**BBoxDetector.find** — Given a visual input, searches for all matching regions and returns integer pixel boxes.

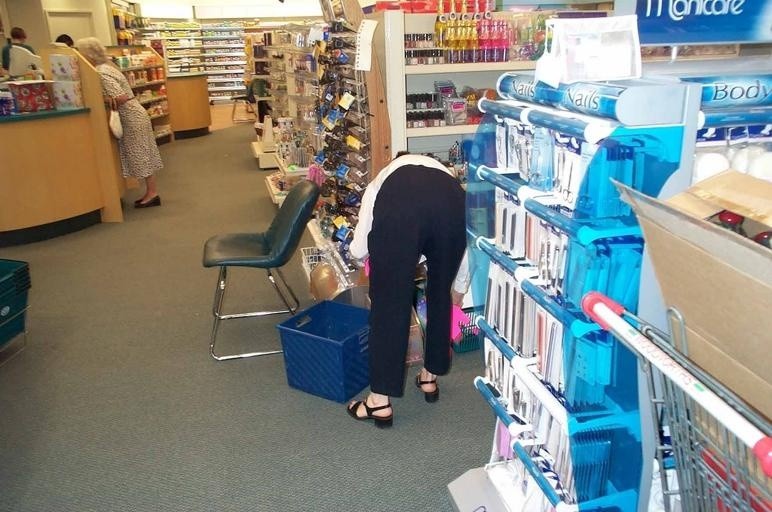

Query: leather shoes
[134,194,160,209]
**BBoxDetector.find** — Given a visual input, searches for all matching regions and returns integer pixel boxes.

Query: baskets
[0,258,32,346]
[300,246,323,282]
[451,305,484,354]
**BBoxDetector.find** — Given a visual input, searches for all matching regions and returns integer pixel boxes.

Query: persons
[1,27,38,70]
[56,34,74,48]
[342,148,468,430]
[74,37,165,208]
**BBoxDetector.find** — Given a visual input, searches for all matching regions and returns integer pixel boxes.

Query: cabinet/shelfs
[110,1,245,148]
[252,0,391,274]
[446,71,703,512]
[365,11,636,193]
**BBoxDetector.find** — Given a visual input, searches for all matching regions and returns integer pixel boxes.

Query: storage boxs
[609,166,772,492]
[2,80,58,113]
[364,293,424,368]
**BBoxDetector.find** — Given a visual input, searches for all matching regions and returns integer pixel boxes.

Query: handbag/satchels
[108,110,123,139]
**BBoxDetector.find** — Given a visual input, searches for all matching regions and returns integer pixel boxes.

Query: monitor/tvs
[9,45,41,76]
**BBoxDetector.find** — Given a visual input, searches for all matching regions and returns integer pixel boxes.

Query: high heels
[415,372,440,403]
[347,398,394,430]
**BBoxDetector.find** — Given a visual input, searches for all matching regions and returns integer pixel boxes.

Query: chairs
[201,179,321,362]
[230,76,257,121]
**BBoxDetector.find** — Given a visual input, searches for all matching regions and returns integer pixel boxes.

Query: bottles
[437,20,510,63]
